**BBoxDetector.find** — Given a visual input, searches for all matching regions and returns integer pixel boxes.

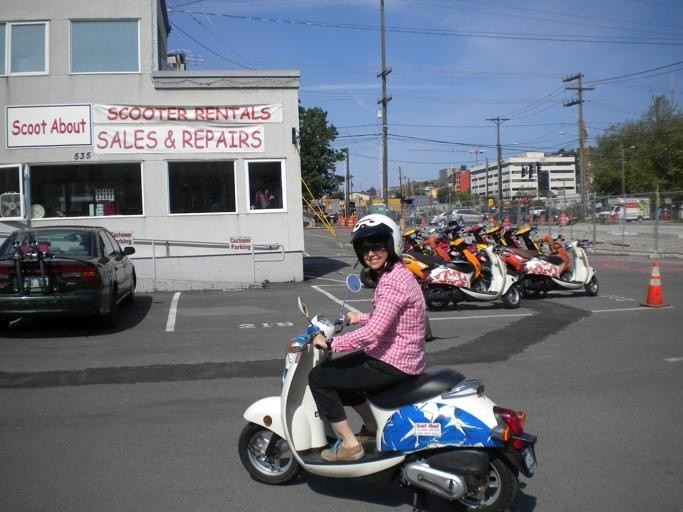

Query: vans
[595,205,642,222]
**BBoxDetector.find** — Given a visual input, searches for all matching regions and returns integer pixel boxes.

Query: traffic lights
[537,163,541,173]
[529,165,533,179]
[521,167,526,177]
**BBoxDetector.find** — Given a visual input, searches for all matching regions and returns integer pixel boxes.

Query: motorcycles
[237,275,538,512]
[359,214,599,309]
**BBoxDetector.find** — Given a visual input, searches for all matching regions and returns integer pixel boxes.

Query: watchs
[324,336,333,350]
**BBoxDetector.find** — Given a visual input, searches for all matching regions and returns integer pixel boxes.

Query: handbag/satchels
[426,316,432,341]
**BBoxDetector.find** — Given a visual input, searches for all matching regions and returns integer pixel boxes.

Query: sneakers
[321,424,376,462]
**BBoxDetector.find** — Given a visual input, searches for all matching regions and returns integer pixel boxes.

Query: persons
[304,211,431,463]
[263,188,275,209]
[250,178,270,210]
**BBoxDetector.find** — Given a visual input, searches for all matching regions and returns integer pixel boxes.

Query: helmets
[351,213,402,268]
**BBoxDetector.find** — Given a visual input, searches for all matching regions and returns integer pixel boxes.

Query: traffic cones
[639,260,670,308]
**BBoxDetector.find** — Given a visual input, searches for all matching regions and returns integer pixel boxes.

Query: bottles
[88,199,118,216]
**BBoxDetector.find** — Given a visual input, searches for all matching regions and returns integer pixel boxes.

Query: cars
[430,212,449,228]
[449,209,487,224]
[529,206,545,216]
[0,225,136,329]
